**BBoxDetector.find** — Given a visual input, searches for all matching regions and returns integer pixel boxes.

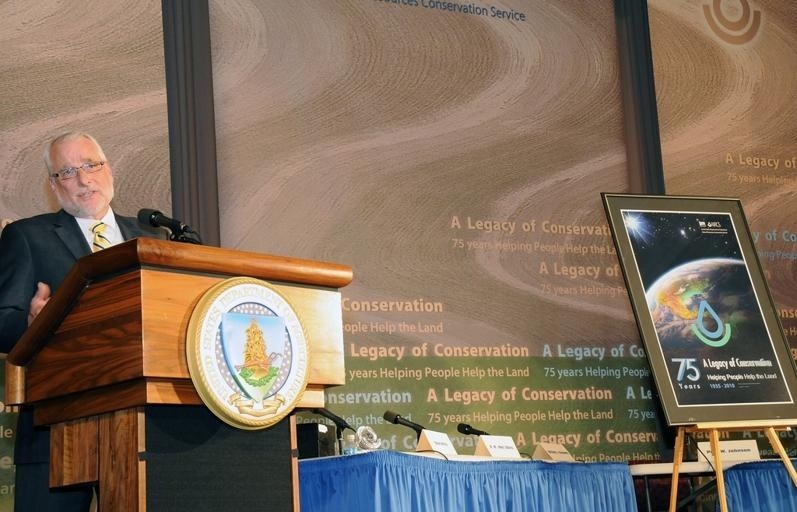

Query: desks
[629,458,796,511]
[298,451,636,512]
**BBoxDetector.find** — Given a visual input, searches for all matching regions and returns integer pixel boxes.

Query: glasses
[51,162,106,180]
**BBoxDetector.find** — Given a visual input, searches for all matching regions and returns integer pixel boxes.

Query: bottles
[343,434,358,456]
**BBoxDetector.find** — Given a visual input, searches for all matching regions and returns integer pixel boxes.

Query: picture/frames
[602,192,795,425]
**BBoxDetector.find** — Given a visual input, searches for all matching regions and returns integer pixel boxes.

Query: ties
[89,220,112,253]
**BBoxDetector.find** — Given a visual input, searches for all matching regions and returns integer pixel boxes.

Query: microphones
[383,411,426,433]
[457,423,491,436]
[309,408,351,429]
[137,209,193,233]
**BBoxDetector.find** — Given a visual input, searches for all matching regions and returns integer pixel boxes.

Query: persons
[0,132,169,511]
